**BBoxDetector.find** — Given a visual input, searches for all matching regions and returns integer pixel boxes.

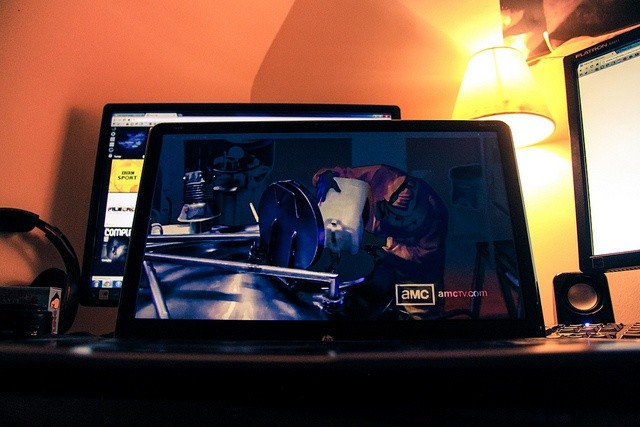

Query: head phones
[1,207,81,335]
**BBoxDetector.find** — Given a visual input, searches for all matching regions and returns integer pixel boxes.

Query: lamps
[451,44,558,146]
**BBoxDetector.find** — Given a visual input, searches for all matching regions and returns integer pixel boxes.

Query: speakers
[553,273,614,325]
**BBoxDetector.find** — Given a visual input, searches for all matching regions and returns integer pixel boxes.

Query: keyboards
[546,322,640,341]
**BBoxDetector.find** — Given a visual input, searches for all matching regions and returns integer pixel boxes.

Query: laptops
[94,120,545,350]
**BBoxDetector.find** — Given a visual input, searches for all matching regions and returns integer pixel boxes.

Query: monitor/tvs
[81,102,400,307]
[563,24,639,273]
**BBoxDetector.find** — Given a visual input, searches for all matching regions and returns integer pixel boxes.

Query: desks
[1,325,603,426]
